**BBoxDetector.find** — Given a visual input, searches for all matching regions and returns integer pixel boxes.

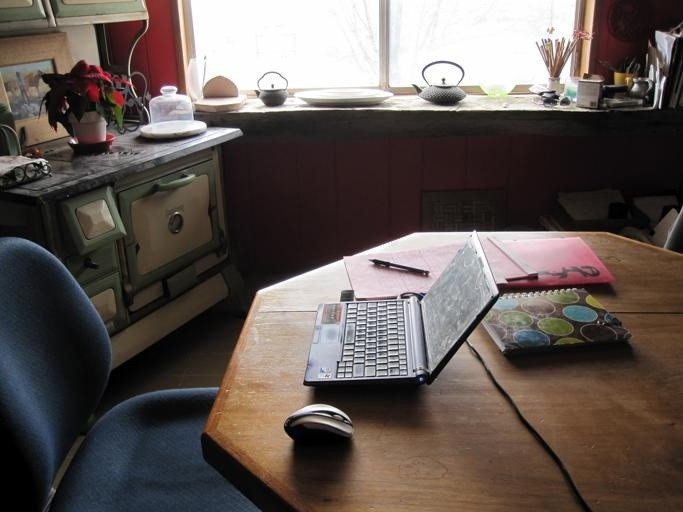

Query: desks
[201,230,682,511]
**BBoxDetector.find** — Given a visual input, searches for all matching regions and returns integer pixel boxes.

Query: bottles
[0,103,19,157]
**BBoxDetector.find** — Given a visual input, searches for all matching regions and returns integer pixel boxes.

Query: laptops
[303,230,500,386]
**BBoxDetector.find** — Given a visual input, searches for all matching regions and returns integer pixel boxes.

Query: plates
[292,87,395,107]
[137,120,207,140]
[66,134,116,153]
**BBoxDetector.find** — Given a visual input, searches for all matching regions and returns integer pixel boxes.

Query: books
[646,29,683,110]
[481,287,632,356]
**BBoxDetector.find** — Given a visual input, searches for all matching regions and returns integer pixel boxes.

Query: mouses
[283,403,354,441]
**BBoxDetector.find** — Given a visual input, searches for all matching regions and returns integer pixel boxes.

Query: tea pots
[253,71,288,108]
[412,60,467,105]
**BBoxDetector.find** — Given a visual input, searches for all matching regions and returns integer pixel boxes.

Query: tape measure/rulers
[487,235,539,276]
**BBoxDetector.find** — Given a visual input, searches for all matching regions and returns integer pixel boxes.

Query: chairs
[0,238,263,510]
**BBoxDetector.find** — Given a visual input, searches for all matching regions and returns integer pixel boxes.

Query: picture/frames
[0,32,82,148]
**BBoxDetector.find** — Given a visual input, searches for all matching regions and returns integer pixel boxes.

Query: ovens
[113,146,248,351]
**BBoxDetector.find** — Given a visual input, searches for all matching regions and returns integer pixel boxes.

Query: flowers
[39,59,133,133]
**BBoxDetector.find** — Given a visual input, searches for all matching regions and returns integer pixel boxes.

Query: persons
[15,71,30,103]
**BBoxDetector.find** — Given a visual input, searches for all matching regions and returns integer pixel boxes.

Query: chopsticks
[535,36,580,77]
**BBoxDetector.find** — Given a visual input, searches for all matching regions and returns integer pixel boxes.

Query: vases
[67,109,107,144]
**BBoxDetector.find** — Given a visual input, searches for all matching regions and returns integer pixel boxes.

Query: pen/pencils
[598,55,641,74]
[536,37,580,77]
[368,259,430,276]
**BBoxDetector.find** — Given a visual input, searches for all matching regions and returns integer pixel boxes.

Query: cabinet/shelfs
[0,0,152,32]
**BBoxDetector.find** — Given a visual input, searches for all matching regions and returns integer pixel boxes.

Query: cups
[614,71,639,91]
[630,76,654,98]
[547,76,559,90]
